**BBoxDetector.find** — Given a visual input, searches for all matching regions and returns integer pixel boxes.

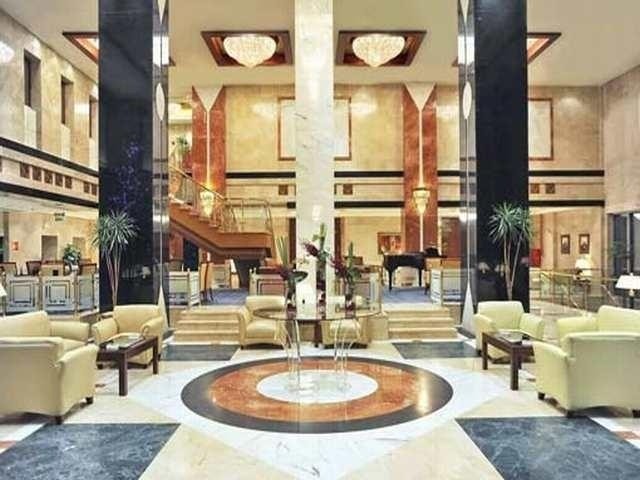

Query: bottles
[100,343,128,351]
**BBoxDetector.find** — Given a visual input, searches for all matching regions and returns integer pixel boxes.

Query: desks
[253,303,382,396]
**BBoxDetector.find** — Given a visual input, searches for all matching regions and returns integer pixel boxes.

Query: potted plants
[335,223,362,312]
[272,232,308,311]
[299,223,334,299]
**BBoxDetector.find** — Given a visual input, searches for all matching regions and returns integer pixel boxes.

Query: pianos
[380,247,446,292]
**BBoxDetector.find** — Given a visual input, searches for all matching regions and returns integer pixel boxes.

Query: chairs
[473,300,545,363]
[237,295,288,350]
[198,262,208,301]
[529,333,640,419]
[319,295,371,349]
[0,310,98,426]
[1,258,97,284]
[90,304,165,369]
[556,304,640,347]
[423,257,462,295]
[205,262,215,302]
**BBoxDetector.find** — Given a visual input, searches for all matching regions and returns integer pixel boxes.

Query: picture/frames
[578,233,589,253]
[377,232,402,254]
[561,235,570,254]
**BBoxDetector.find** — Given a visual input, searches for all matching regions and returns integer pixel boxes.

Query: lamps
[351,33,405,69]
[411,186,430,214]
[222,34,276,71]
[199,186,218,217]
